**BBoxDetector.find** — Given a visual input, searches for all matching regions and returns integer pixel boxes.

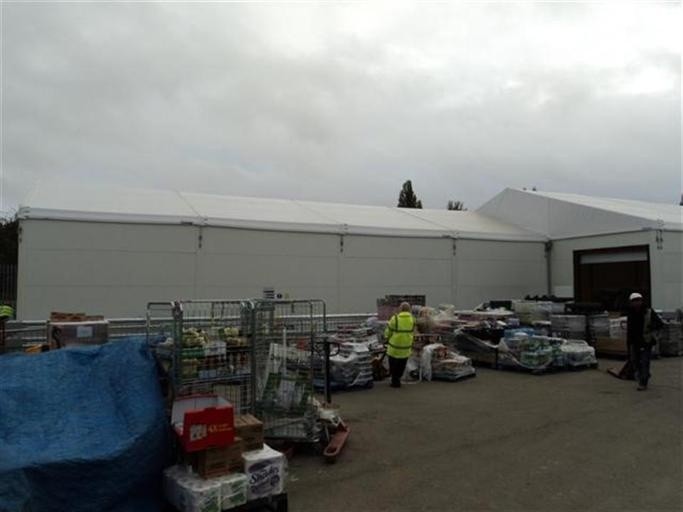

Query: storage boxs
[187,436,245,479]
[551,315,586,332]
[48,318,108,350]
[233,415,263,450]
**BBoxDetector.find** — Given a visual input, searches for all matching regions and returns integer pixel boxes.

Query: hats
[628,292,642,300]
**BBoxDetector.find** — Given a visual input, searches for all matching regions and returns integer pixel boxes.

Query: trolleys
[369,336,388,382]
[606,320,638,381]
[277,340,350,464]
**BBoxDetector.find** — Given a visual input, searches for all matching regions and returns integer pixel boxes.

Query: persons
[627,292,664,391]
[0,300,15,320]
[383,301,417,388]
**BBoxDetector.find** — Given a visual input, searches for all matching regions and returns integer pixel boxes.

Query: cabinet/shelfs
[147,299,255,415]
